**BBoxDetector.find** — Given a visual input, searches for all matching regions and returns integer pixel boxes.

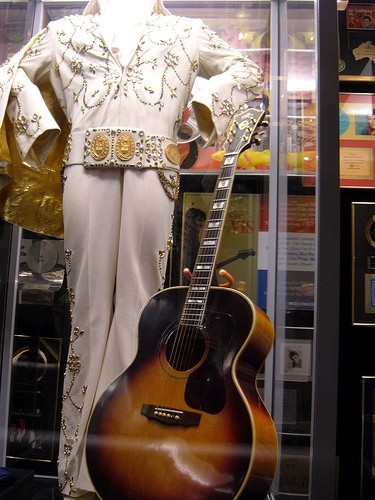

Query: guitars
[86,103,280,500]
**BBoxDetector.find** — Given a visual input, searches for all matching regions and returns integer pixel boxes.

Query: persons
[182,209,208,286]
[289,351,302,368]
[0,2,264,499]
[359,15,372,29]
[361,109,375,135]
